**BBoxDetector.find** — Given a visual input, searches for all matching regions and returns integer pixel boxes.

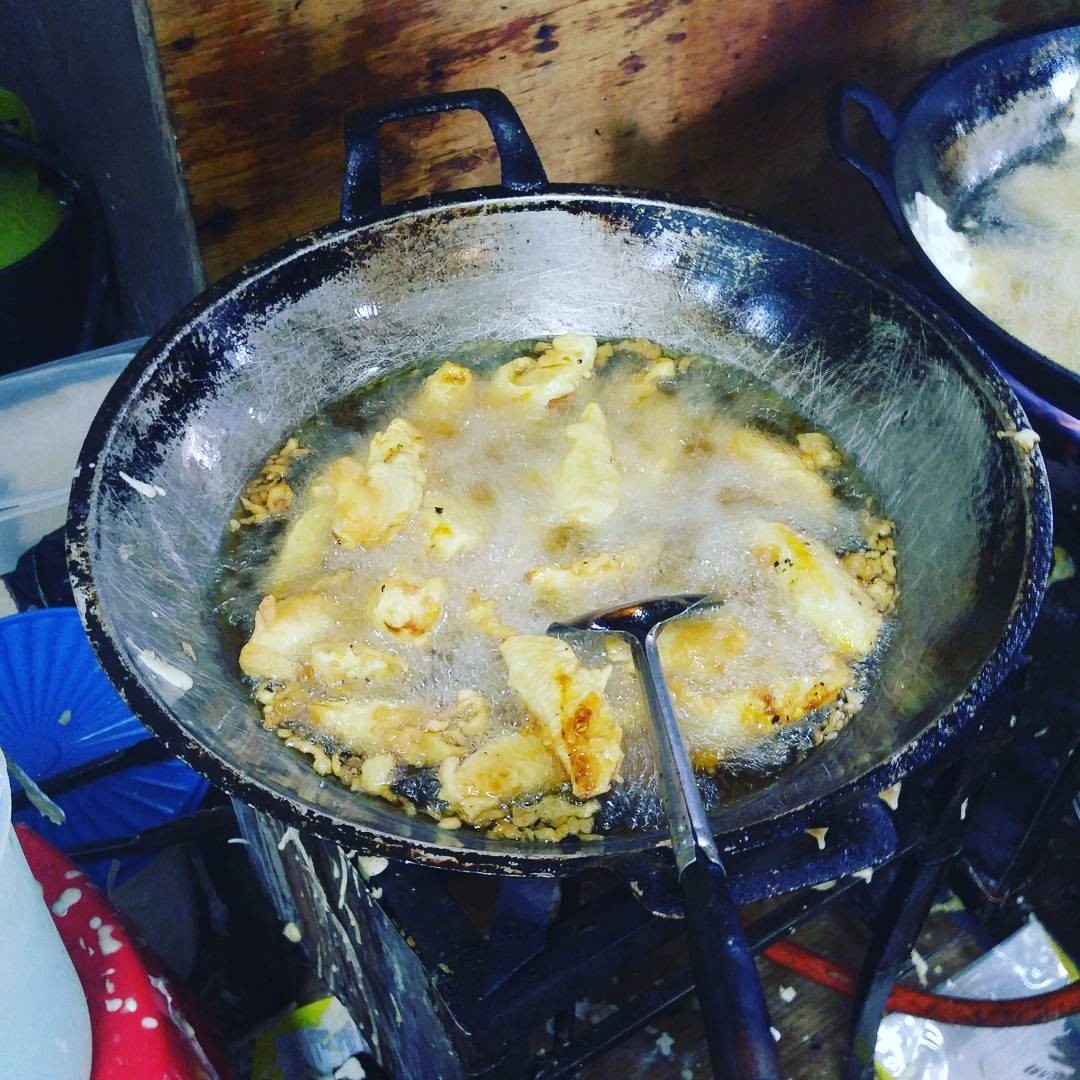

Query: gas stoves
[221,255,1080,1080]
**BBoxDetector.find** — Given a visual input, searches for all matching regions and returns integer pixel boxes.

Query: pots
[62,86,1052,923]
[829,27,1078,427]
